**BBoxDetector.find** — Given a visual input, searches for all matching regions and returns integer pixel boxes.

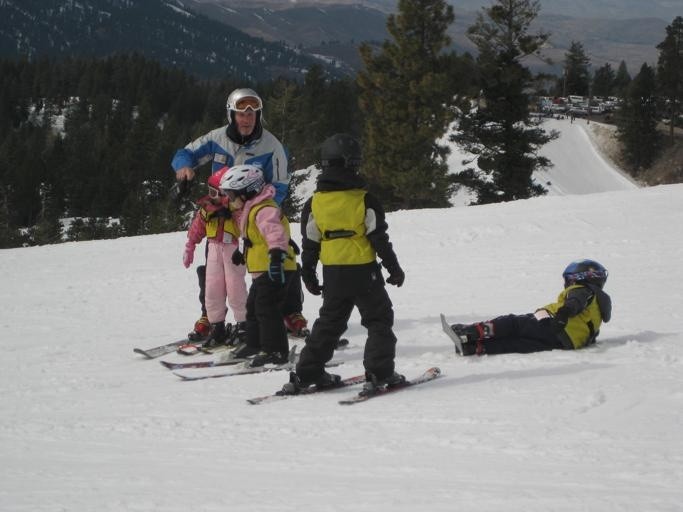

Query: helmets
[226,88,263,124]
[219,165,266,210]
[320,134,364,175]
[562,260,608,289]
[208,166,230,207]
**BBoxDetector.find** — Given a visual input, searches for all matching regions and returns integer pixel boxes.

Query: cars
[530,95,625,117]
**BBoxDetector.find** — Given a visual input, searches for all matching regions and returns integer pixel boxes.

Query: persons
[171,87,307,340]
[183,166,248,349]
[219,164,299,368]
[296,133,408,391]
[451,259,612,356]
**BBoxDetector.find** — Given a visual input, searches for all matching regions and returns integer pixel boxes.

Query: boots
[451,321,490,355]
[206,322,227,345]
[225,322,247,346]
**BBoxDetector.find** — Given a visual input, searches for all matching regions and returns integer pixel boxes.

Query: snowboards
[441,312,468,357]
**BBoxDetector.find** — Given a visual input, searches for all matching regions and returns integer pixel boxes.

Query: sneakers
[283,311,307,333]
[195,317,210,337]
[296,364,336,387]
[250,351,289,367]
[230,342,261,359]
[365,370,401,391]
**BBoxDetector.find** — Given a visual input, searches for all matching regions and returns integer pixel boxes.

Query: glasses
[208,185,223,200]
[234,96,263,112]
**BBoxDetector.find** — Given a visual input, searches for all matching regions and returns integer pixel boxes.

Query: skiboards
[247,367,441,405]
[133,332,347,383]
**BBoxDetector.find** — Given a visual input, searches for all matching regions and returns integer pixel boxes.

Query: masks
[229,195,246,210]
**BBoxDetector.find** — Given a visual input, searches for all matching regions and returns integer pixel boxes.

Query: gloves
[382,254,404,287]
[301,269,323,295]
[267,248,287,285]
[170,175,197,200]
[553,306,570,332]
[183,243,195,268]
